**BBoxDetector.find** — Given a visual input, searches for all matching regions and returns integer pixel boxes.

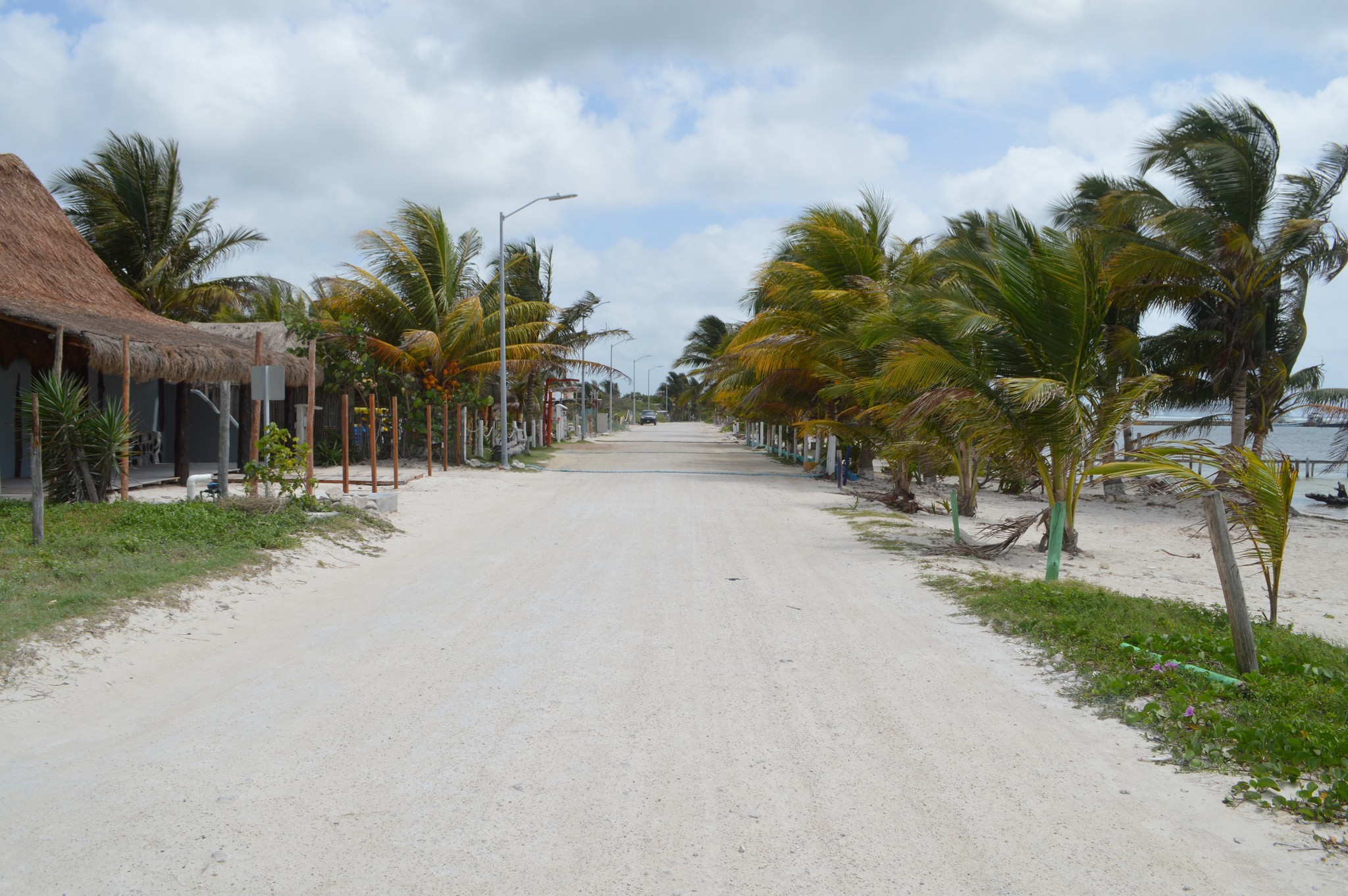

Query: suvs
[639,410,658,425]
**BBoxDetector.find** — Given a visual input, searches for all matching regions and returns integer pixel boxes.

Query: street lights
[495,191,580,470]
[632,354,653,424]
[581,300,611,441]
[647,366,664,409]
[609,337,637,424]
